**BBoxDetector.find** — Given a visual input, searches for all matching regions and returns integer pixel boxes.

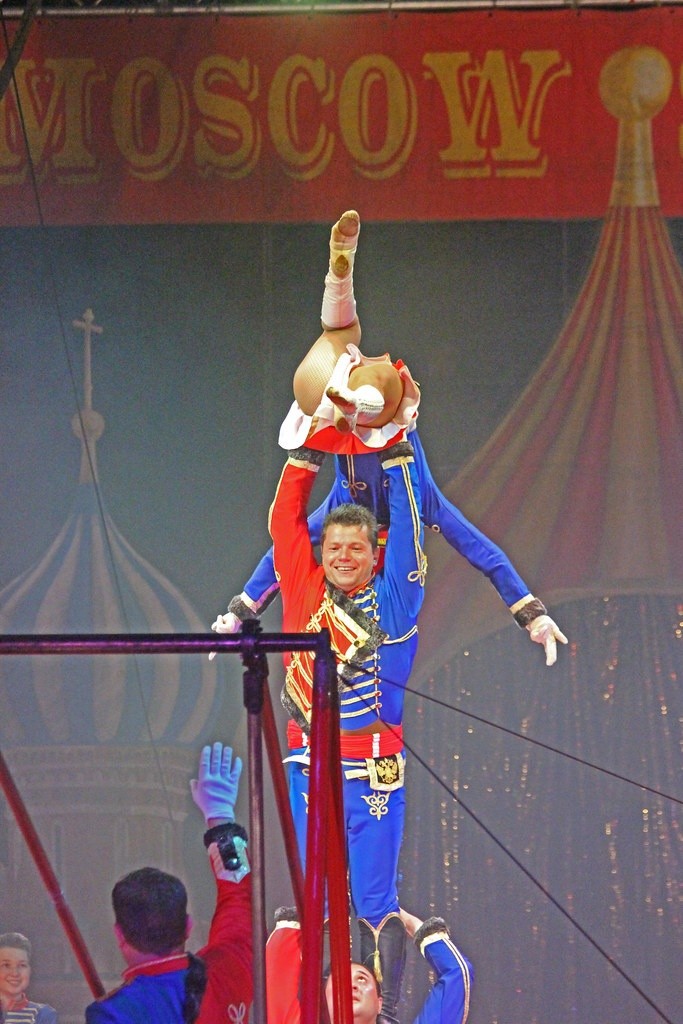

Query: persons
[204,210,573,670]
[267,426,472,1024]
[82,742,253,1024]
[0,930,58,1024]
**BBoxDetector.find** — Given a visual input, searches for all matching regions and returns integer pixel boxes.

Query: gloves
[526,614,569,667]
[207,611,242,661]
[189,741,243,823]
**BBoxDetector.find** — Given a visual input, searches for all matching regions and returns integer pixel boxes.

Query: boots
[297,915,352,999]
[357,912,407,1024]
